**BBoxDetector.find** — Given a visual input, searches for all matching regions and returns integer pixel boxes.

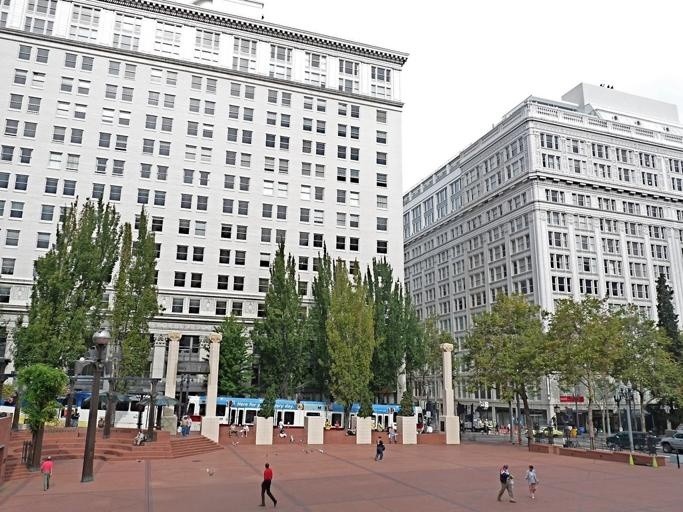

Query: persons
[459,421,463,441]
[546,416,555,445]
[132,430,145,446]
[323,418,332,431]
[239,424,250,438]
[228,422,239,438]
[482,418,489,435]
[388,425,397,443]
[39,455,54,492]
[333,416,339,430]
[279,427,287,442]
[372,436,385,459]
[69,408,80,427]
[523,464,539,500]
[374,440,385,462]
[257,462,277,508]
[180,417,188,437]
[186,415,192,436]
[569,426,578,449]
[278,419,284,430]
[496,464,516,503]
[416,422,424,434]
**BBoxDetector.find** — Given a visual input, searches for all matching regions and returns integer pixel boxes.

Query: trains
[184,394,426,434]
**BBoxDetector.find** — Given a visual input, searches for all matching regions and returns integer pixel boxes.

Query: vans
[77,395,163,429]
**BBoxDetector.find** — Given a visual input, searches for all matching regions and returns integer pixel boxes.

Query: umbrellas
[135,393,180,408]
[82,390,131,426]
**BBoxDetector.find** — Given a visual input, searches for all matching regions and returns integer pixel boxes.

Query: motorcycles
[649,438,657,454]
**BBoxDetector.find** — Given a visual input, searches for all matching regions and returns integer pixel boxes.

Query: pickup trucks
[526,425,564,437]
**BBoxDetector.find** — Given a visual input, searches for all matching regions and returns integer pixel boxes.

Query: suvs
[606,431,648,450]
[661,432,683,453]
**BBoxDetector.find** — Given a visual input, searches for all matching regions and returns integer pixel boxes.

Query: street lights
[554,405,560,425]
[614,392,623,431]
[81,329,111,481]
[621,386,635,452]
